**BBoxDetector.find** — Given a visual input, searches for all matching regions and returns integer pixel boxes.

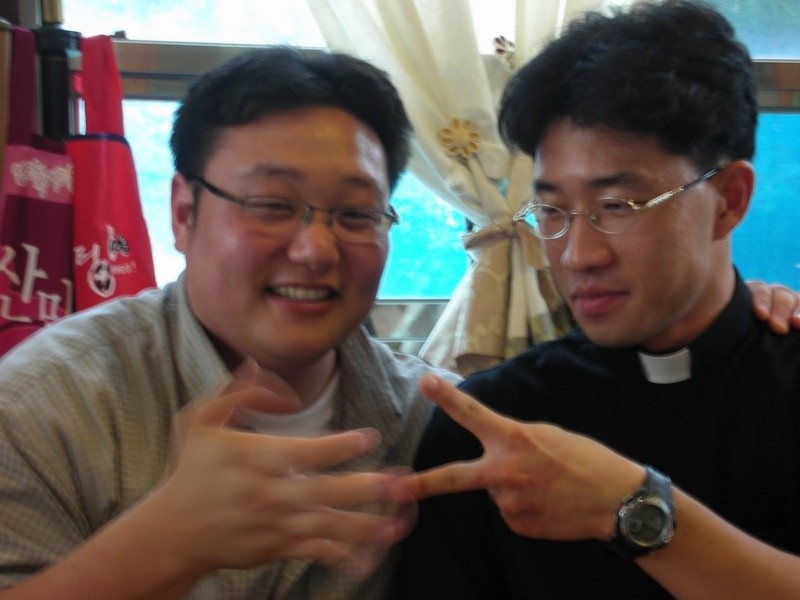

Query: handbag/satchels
[64,31,159,312]
[0,26,74,357]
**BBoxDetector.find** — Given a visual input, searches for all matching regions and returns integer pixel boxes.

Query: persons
[318,1,800,597]
[0,42,800,598]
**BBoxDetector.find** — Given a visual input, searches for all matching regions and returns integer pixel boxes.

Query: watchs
[608,464,679,574]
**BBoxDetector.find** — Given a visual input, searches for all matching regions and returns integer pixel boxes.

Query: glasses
[510,158,728,242]
[186,173,400,246]
